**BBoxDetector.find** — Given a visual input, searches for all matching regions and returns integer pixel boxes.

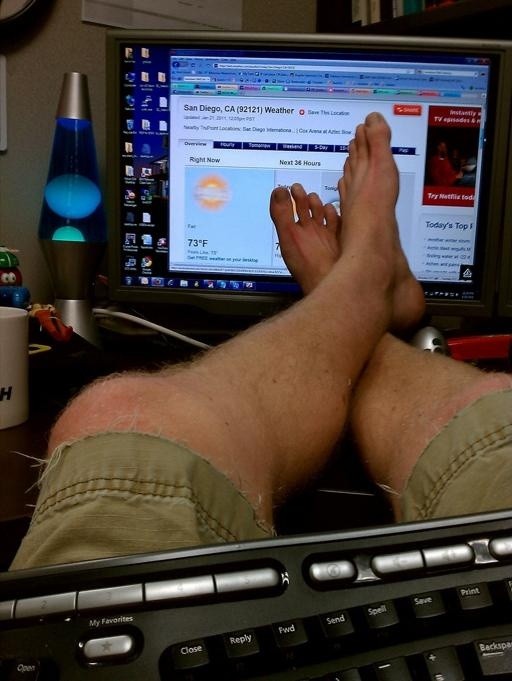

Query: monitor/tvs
[103,27,512,328]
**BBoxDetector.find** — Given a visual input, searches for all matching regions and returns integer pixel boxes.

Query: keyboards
[0,487,512,681]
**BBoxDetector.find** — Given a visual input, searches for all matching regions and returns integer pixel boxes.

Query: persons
[1,110,511,575]
